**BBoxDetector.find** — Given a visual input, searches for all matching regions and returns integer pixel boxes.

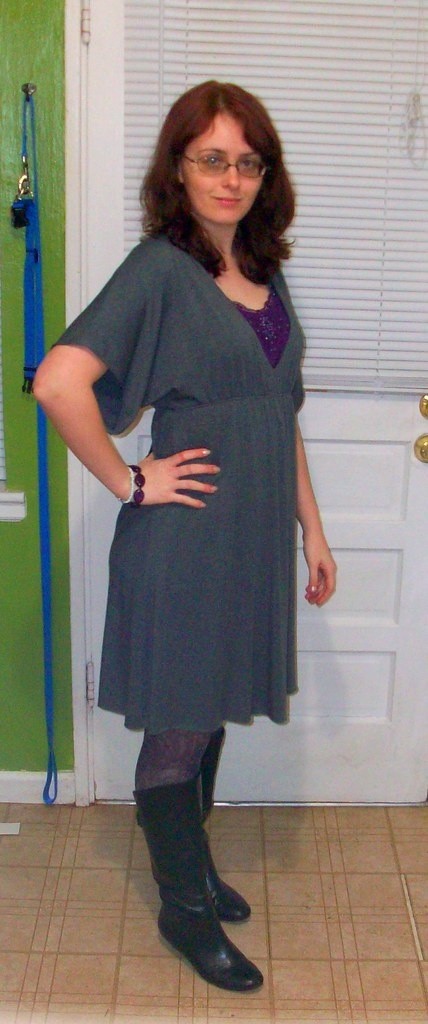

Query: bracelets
[122,463,147,506]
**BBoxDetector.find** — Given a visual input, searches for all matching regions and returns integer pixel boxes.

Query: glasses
[181,154,267,178]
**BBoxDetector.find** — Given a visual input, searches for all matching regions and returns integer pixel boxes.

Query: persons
[32,80,338,995]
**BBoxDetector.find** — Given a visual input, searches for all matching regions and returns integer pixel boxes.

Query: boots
[132,771,264,992]
[135,728,251,924]
